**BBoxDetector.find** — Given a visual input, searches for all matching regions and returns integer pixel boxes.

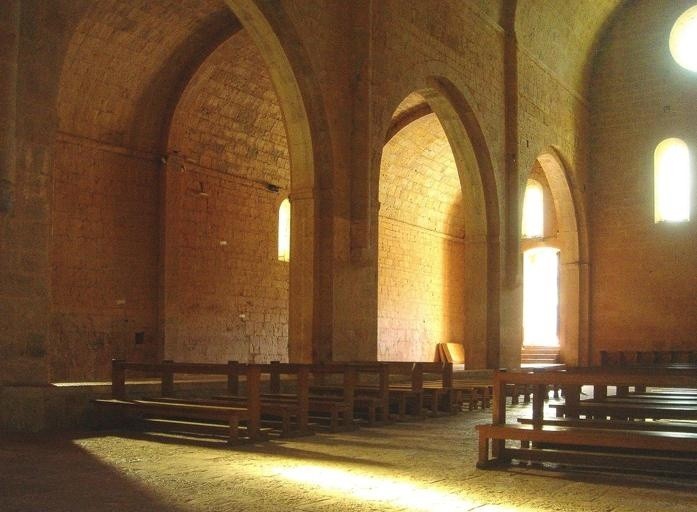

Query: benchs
[89,358,696,475]
